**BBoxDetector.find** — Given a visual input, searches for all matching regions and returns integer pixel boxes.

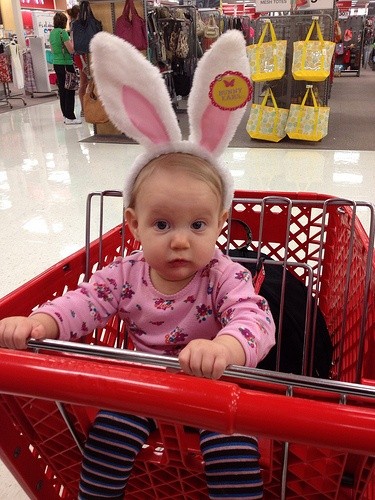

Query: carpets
[0,89,58,114]
[79,68,375,151]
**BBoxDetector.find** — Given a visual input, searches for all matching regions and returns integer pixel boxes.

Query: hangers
[0,36,21,47]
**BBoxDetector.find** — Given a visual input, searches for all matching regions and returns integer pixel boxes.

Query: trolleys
[0,189,375,500]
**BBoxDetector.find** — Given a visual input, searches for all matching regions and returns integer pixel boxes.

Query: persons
[49,12,82,124]
[0,141,276,500]
[66,5,97,116]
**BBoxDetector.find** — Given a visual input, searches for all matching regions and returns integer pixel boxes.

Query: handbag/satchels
[291,19,336,83]
[82,79,109,125]
[195,11,205,37]
[72,1,102,56]
[65,71,79,91]
[285,88,331,143]
[246,22,287,84]
[115,1,148,52]
[216,250,335,377]
[203,14,219,40]
[246,88,289,143]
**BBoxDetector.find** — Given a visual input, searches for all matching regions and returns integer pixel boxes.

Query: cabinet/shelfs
[77,0,375,138]
[0,0,375,143]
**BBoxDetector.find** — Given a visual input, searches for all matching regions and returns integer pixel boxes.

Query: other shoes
[65,117,82,124]
